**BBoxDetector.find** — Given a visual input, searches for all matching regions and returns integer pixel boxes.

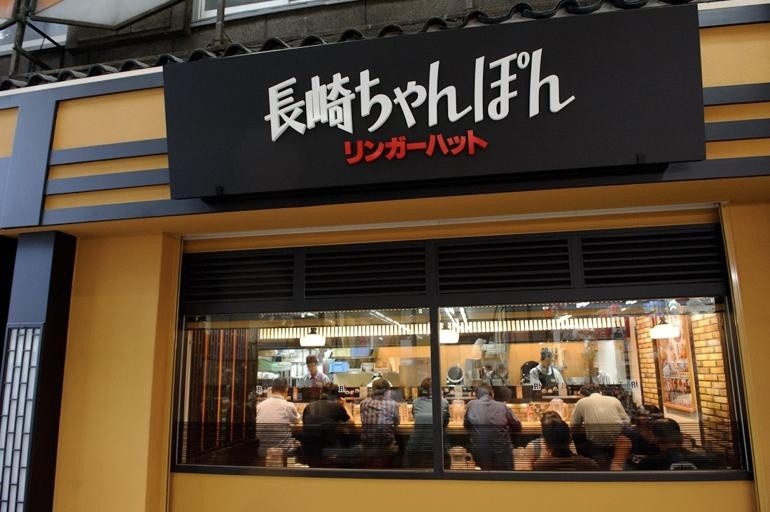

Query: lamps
[649,309,679,339]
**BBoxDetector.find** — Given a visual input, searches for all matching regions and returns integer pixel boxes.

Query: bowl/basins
[469,379,483,386]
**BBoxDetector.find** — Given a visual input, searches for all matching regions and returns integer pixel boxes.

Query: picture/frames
[653,314,697,415]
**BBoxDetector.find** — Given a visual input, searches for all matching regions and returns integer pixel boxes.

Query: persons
[256,378,449,470]
[303,356,331,387]
[525,384,711,471]
[463,384,523,470]
[530,348,565,396]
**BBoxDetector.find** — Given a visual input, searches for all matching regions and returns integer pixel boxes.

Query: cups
[265,447,283,467]
[549,397,569,423]
[452,399,464,426]
[411,384,419,400]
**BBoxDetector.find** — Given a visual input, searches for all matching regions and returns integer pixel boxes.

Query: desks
[293,403,632,434]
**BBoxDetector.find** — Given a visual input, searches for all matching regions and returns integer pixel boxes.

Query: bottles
[447,445,473,470]
[511,446,532,470]
